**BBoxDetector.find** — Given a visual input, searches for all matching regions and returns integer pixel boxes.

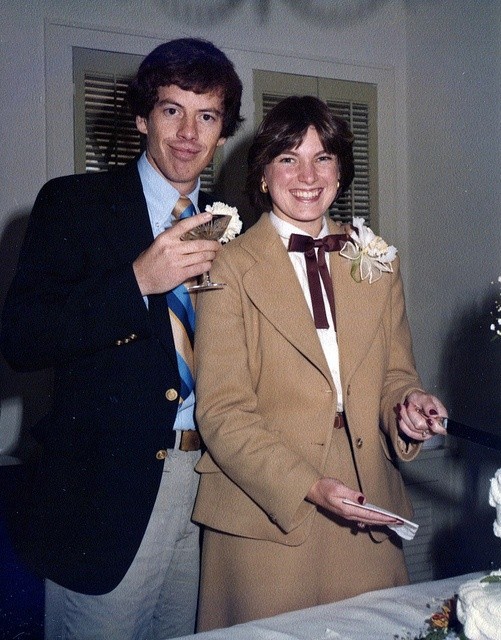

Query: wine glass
[171,212,233,294]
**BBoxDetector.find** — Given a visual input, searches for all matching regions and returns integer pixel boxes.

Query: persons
[1,36,245,633]
[195,94,438,629]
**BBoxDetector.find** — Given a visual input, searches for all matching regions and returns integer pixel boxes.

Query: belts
[170,428,203,452]
[329,414,347,428]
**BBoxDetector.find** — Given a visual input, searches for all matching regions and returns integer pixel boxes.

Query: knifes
[418,399,500,453]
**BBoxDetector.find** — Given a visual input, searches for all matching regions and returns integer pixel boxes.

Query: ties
[167,197,199,404]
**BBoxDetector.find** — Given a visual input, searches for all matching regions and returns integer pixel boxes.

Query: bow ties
[287,233,353,332]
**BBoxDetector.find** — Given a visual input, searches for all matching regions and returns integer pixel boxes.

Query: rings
[422,431,427,437]
